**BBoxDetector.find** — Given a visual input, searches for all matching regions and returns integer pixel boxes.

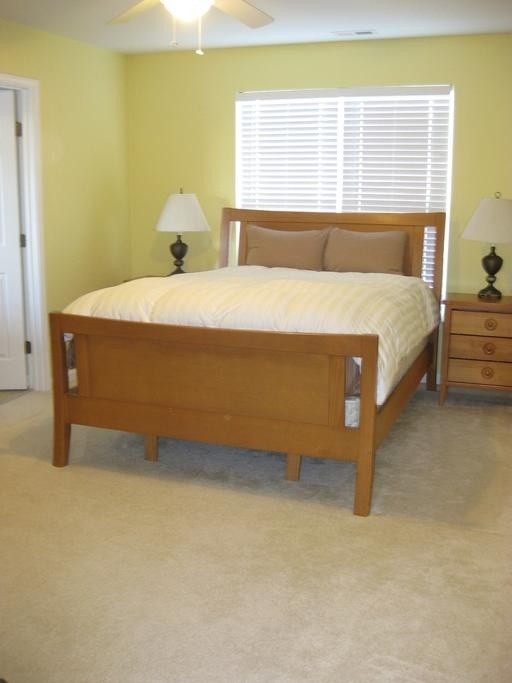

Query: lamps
[458,191,512,300]
[151,186,212,277]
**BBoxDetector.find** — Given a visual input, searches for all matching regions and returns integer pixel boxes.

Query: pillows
[241,223,409,276]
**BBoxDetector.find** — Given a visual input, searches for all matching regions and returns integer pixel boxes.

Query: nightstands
[437,291,512,407]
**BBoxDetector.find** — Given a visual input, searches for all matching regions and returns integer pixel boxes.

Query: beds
[46,206,447,517]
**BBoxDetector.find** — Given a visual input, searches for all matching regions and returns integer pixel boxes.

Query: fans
[101,0,276,32]
